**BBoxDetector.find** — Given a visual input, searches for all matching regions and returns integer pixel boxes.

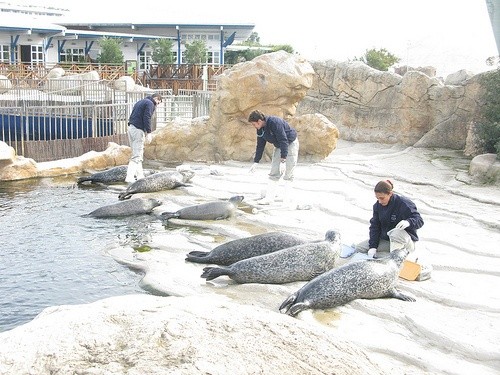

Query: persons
[248,111,300,206]
[366,179,424,259]
[125,92,162,187]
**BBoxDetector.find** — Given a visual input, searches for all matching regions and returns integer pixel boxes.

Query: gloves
[396,220,410,230]
[247,163,259,174]
[280,162,286,174]
[368,248,377,258]
[146,132,153,144]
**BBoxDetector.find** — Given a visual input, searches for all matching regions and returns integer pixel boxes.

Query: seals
[77,164,156,185]
[82,196,164,218]
[181,228,345,284]
[278,246,416,317]
[162,195,246,221]
[119,169,196,201]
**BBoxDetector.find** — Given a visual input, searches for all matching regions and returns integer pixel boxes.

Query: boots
[125,161,138,183]
[257,180,279,205]
[136,163,145,180]
[282,180,294,208]
